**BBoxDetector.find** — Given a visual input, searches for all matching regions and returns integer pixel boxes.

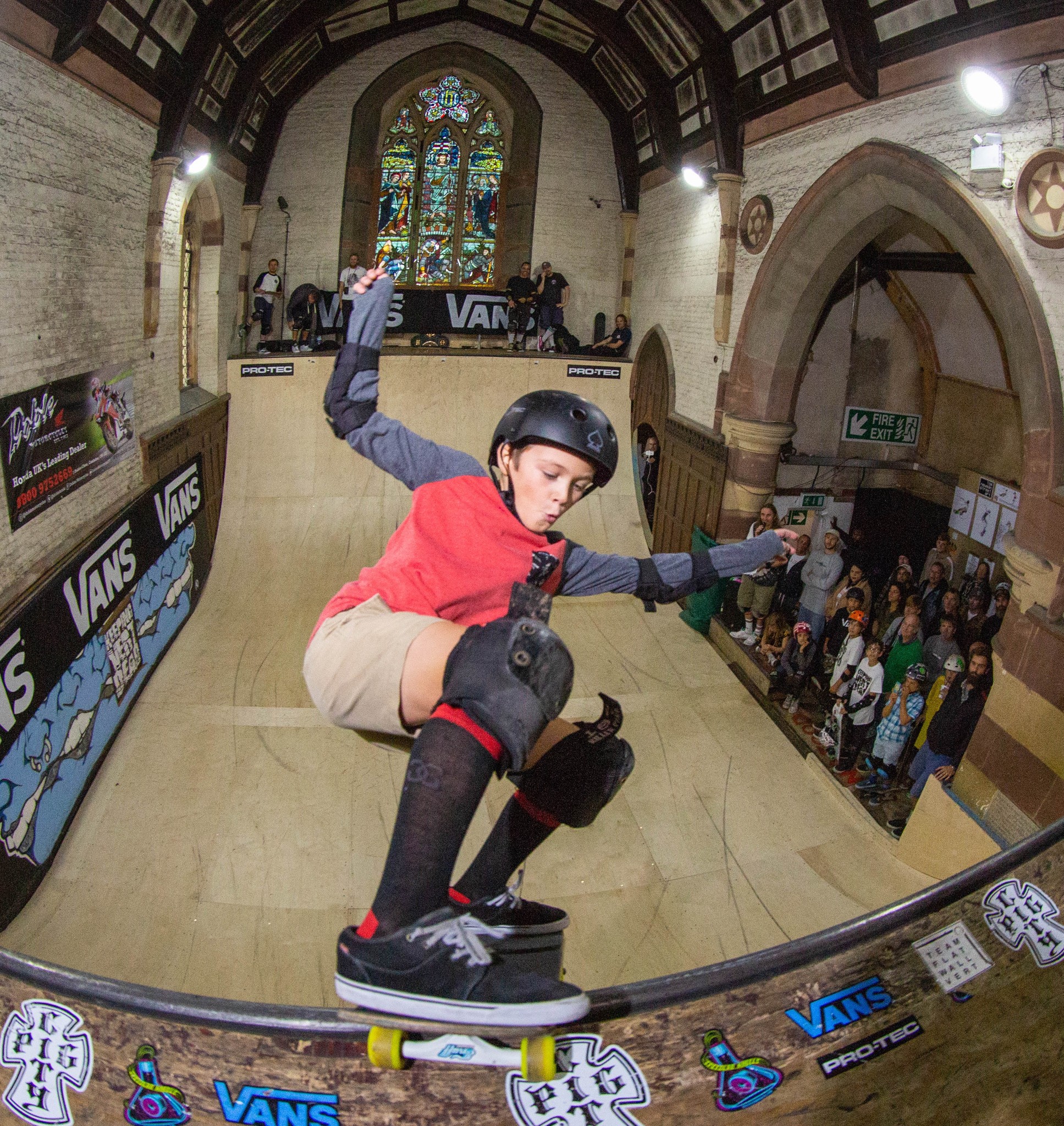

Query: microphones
[758,522,766,535]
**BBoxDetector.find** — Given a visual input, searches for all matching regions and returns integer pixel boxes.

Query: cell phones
[646,451,654,455]
[893,681,901,694]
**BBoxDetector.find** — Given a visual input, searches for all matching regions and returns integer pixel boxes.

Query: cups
[938,685,949,700]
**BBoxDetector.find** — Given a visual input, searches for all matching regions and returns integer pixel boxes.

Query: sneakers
[446,869,570,935]
[335,907,591,1026]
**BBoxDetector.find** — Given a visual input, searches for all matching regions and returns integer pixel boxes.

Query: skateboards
[335,1004,558,1084]
[825,701,846,762]
[256,339,294,352]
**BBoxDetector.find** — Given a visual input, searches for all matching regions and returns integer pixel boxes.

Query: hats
[826,529,840,538]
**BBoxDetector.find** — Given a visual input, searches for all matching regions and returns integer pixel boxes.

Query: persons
[237,253,632,356]
[303,269,801,1022]
[90,378,131,423]
[640,436,1012,839]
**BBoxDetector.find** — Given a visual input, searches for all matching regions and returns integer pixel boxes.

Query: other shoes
[812,722,871,774]
[729,629,801,714]
[854,774,906,838]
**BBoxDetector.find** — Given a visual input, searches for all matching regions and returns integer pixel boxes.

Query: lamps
[961,63,1047,117]
[971,133,1004,171]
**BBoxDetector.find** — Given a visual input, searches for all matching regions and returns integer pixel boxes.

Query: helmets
[793,622,811,639]
[905,662,929,683]
[943,655,966,672]
[488,390,619,488]
[848,610,869,626]
[846,587,865,603]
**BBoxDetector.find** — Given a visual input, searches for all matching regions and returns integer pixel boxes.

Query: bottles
[317,335,322,345]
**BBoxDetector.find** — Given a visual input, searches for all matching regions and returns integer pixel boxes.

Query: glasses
[869,647,880,652]
